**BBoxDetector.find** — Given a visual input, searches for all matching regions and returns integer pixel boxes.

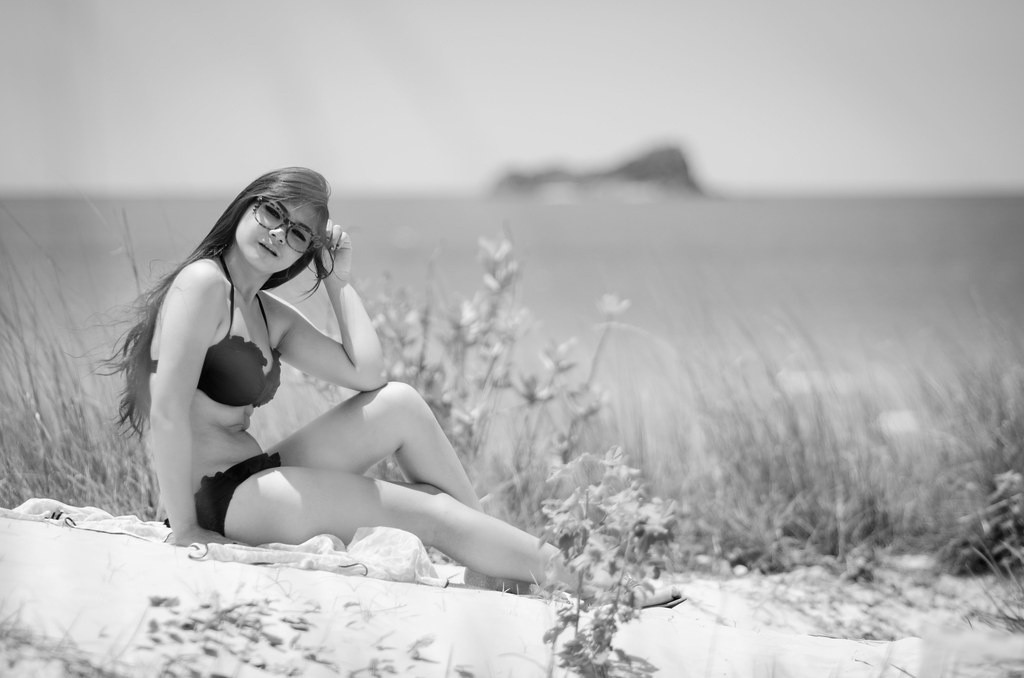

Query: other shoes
[583,578,687,608]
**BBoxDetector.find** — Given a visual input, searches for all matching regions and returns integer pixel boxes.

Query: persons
[100,167,691,610]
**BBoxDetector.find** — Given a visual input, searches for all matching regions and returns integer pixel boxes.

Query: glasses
[252,196,324,254]
